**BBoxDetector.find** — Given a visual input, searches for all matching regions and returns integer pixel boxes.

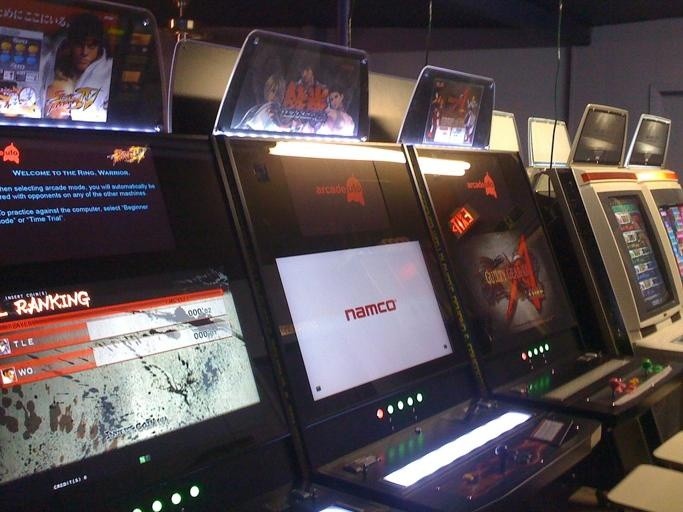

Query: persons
[236,62,354,136]
[42,15,114,124]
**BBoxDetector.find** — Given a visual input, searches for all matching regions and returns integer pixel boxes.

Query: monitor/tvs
[661,203,683,282]
[227,136,473,428]
[608,194,675,311]
[408,148,572,360]
[0,140,264,511]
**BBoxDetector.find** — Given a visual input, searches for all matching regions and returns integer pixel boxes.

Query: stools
[608,465,682,512]
[652,429,683,465]
